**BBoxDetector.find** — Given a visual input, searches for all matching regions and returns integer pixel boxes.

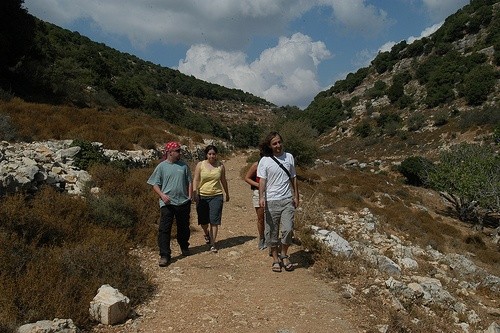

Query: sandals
[279,255,294,271]
[272,260,282,272]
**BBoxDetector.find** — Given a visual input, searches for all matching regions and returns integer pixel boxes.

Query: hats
[166,141,180,151]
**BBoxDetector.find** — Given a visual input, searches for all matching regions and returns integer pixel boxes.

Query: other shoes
[205,234,210,243]
[181,246,190,256]
[159,255,170,267]
[258,236,265,248]
[210,245,217,252]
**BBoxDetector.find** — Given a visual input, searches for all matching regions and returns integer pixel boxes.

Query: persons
[146,142,193,266]
[192,145,230,253]
[257,131,299,273]
[245,149,268,250]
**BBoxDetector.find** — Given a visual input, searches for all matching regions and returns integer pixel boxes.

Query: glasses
[169,149,181,152]
